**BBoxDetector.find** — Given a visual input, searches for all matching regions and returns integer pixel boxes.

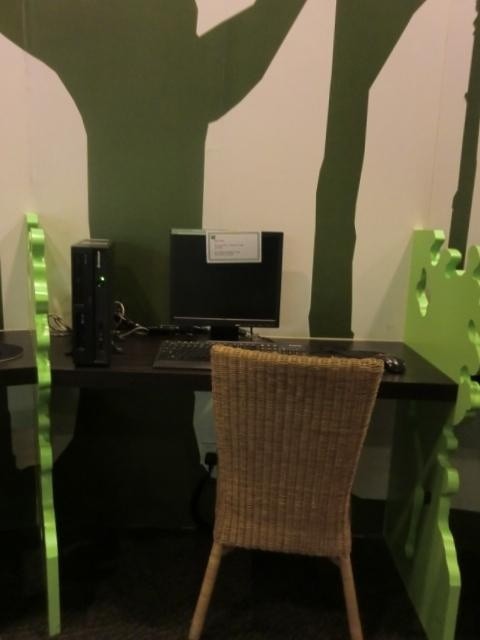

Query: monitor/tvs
[169,228,284,340]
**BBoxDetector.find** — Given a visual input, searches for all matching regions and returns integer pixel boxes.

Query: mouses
[378,355,406,374]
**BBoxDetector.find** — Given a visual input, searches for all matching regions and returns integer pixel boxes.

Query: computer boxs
[71,238,114,368]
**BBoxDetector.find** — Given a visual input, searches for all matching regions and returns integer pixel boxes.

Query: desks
[0,322,461,632]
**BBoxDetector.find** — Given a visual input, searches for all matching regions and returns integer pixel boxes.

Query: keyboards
[152,339,310,369]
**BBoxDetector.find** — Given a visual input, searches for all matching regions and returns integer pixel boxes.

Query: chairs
[189,342,387,640]
